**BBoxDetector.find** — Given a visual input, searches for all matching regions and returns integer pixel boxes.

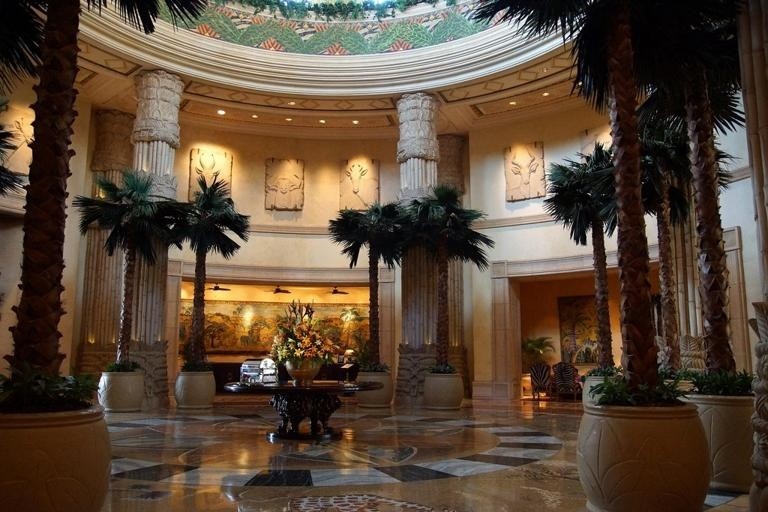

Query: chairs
[551,360,584,402]
[528,360,556,401]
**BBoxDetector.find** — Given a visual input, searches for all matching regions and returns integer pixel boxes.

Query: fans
[322,286,352,296]
[206,283,231,293]
[264,284,292,295]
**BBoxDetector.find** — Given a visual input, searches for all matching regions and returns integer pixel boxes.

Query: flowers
[269,297,331,364]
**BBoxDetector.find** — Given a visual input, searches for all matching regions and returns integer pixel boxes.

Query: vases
[285,359,321,383]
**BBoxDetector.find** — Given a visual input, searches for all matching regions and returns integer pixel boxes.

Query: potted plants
[0,1,207,511]
[148,166,252,410]
[327,199,409,409]
[377,181,495,411]
[70,168,187,414]
[467,2,768,510]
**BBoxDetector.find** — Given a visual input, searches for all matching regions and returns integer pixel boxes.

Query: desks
[223,379,385,445]
